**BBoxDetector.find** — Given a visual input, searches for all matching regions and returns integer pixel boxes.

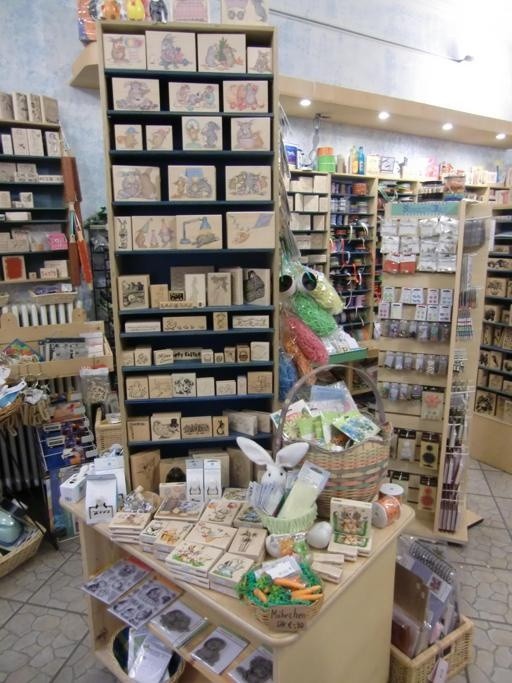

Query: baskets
[272,362,391,520]
[107,622,187,683]
[389,612,475,682]
[243,557,324,633]
[0,516,48,581]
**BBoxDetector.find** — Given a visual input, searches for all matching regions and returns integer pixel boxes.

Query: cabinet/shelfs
[100,24,276,494]
[1,92,110,517]
[289,171,511,530]
[68,502,410,682]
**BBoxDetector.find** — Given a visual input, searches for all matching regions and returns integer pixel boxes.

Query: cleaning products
[349,146,358,174]
[359,146,364,174]
